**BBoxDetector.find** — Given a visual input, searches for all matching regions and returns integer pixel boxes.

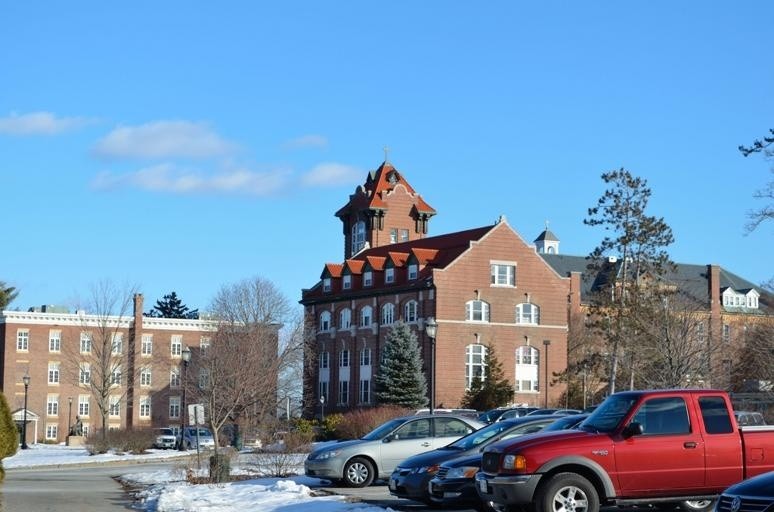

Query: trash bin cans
[209,455,230,483]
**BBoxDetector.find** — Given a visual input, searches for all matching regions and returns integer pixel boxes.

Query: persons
[69,413,83,434]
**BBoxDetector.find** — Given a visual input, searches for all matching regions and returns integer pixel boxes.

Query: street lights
[67,396,73,435]
[178,344,192,449]
[21,369,32,449]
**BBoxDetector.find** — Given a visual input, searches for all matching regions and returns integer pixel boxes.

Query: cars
[153,428,177,450]
[242,438,262,450]
[302,415,484,488]
[713,471,774,512]
[427,412,597,507]
[273,432,288,444]
[416,403,601,425]
[388,414,571,506]
[177,427,214,450]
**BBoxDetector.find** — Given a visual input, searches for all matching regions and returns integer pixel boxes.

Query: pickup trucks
[472,387,774,512]
